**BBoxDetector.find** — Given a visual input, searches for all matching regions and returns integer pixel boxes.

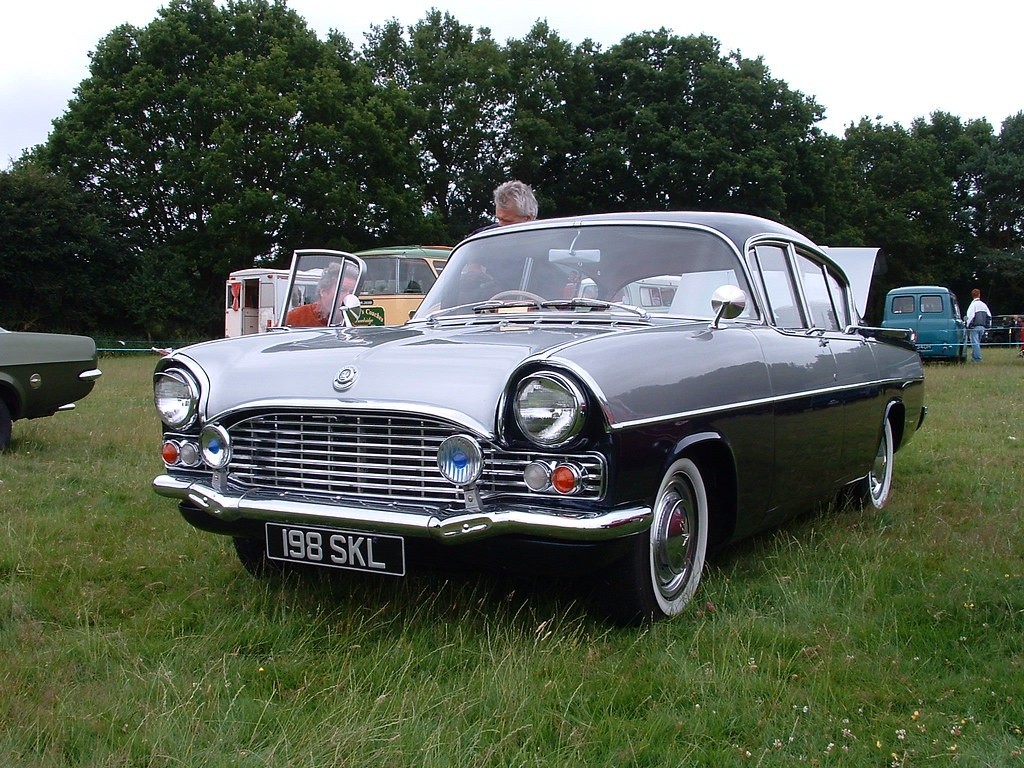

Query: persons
[965,289,992,361]
[1003,314,1024,356]
[274,263,361,327]
[472,181,548,302]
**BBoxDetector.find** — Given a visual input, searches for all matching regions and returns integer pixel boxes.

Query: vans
[881,283,969,363]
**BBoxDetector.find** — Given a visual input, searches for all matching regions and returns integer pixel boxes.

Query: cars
[214,240,604,345]
[152,209,930,625]
[0,324,104,455]
